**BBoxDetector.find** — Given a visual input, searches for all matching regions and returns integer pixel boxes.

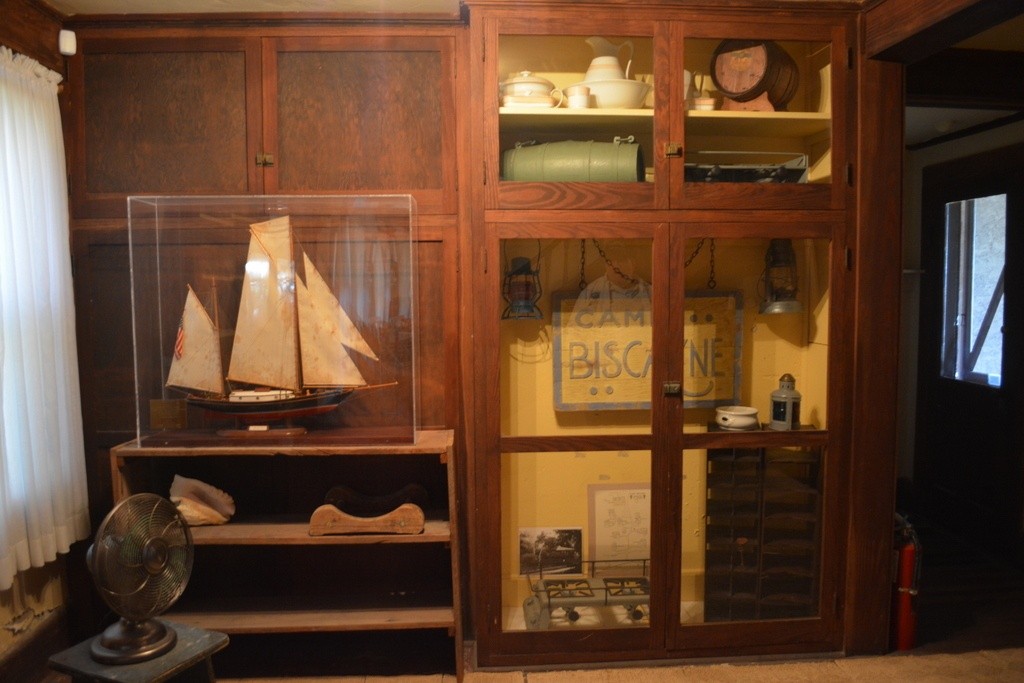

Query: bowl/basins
[716,406,759,431]
[687,97,716,110]
[562,79,650,109]
[503,71,555,96]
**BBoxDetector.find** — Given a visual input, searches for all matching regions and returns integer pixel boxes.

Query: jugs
[583,37,635,80]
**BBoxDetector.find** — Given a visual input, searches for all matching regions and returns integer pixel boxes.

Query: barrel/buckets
[710,37,800,108]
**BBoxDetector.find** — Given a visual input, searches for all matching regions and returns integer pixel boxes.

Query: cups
[502,88,563,108]
[567,86,590,109]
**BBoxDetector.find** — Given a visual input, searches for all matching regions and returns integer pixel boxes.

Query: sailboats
[162,213,399,429]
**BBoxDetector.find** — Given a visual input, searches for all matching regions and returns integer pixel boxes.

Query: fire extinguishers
[891,513,926,652]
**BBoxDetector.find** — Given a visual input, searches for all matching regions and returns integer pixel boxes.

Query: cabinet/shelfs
[49,0,852,683]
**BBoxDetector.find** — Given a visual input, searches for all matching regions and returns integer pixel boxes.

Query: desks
[49,623,229,683]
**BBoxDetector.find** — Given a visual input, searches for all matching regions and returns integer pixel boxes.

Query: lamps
[502,238,545,321]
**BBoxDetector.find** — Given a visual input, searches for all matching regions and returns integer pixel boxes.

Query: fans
[87,493,191,664]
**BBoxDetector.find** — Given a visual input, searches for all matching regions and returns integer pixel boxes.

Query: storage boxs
[125,193,422,450]
